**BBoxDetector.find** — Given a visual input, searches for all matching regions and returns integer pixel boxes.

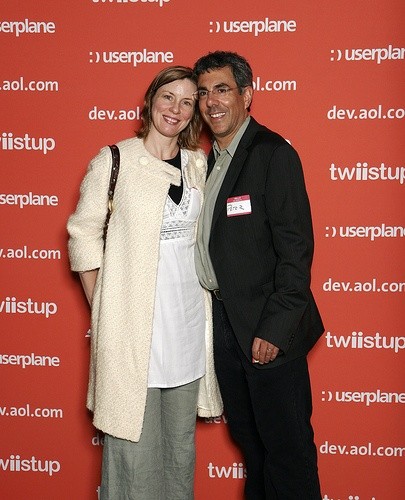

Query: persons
[193,51,325,500]
[66,65,223,500]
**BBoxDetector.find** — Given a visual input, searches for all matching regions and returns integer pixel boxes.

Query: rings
[267,347,274,350]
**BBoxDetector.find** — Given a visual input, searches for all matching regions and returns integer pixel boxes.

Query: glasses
[192,86,246,100]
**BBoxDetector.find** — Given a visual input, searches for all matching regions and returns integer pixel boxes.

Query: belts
[210,289,222,301]
